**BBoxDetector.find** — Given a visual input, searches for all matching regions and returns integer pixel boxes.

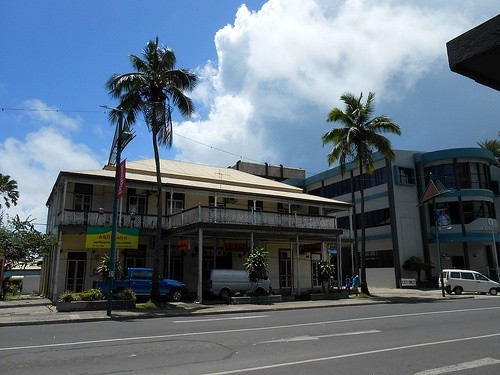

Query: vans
[438,269,500,295]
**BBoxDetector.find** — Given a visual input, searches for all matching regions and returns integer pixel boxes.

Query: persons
[345,275,351,297]
[351,271,360,296]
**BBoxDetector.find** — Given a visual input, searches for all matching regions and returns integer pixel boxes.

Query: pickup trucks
[97,267,187,301]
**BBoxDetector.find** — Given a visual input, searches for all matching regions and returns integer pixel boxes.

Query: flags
[116,160,126,198]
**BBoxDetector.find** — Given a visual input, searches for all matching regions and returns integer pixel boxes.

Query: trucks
[211,268,272,299]
